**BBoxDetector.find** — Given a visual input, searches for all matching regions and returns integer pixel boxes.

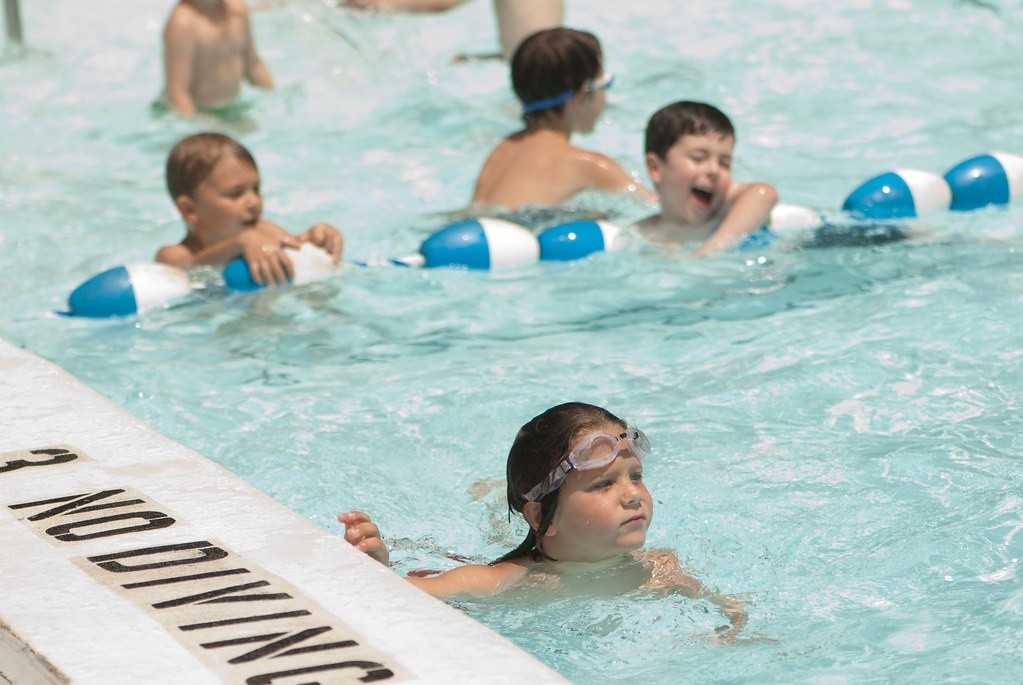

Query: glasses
[523,427,651,502]
[522,73,615,112]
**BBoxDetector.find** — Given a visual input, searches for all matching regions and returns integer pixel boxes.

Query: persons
[336,401,749,646]
[337,0,565,66]
[469,27,662,211]
[629,101,778,259]
[160,0,274,120]
[151,132,344,288]
